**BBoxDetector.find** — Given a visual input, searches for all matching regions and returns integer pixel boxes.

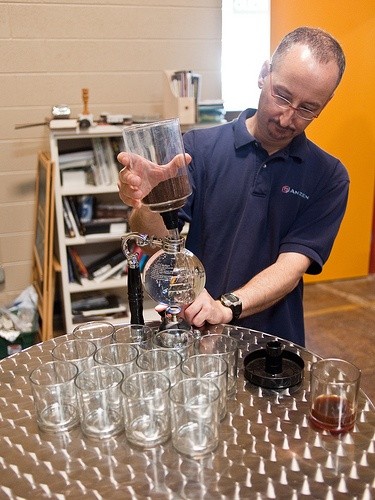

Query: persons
[117,27,350,346]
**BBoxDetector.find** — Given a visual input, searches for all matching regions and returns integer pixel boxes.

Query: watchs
[217,292,242,325]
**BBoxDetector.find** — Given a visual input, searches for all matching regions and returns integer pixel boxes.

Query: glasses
[269,63,330,121]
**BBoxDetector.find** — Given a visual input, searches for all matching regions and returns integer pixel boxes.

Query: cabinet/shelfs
[49,119,161,335]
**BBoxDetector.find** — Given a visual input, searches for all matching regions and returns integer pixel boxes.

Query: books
[171,71,224,122]
[58,137,147,325]
[49,114,80,130]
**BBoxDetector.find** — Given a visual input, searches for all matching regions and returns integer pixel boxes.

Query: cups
[196,333,239,396]
[52,339,97,374]
[166,378,221,458]
[136,348,184,403]
[180,354,230,423]
[29,360,79,435]
[93,343,139,381]
[150,328,196,359]
[120,371,171,448]
[73,320,116,352]
[74,365,125,442]
[308,357,362,436]
[112,324,154,350]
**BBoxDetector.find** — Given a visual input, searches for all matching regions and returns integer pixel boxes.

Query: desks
[0,320,375,500]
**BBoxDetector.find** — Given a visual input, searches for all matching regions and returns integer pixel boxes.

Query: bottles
[122,117,193,241]
